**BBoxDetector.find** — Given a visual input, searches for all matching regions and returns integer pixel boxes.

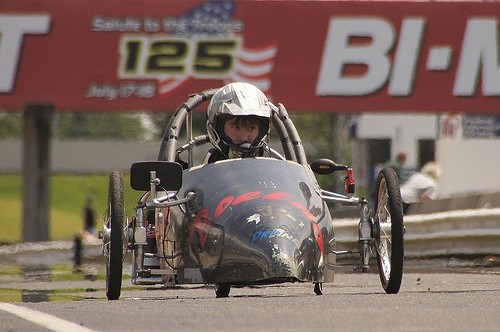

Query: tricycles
[95,83,408,299]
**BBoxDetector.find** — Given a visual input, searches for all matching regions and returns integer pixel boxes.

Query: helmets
[206,82,272,159]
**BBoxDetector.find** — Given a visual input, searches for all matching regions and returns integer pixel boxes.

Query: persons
[384,152,440,214]
[202,82,285,164]
[82,197,98,235]
